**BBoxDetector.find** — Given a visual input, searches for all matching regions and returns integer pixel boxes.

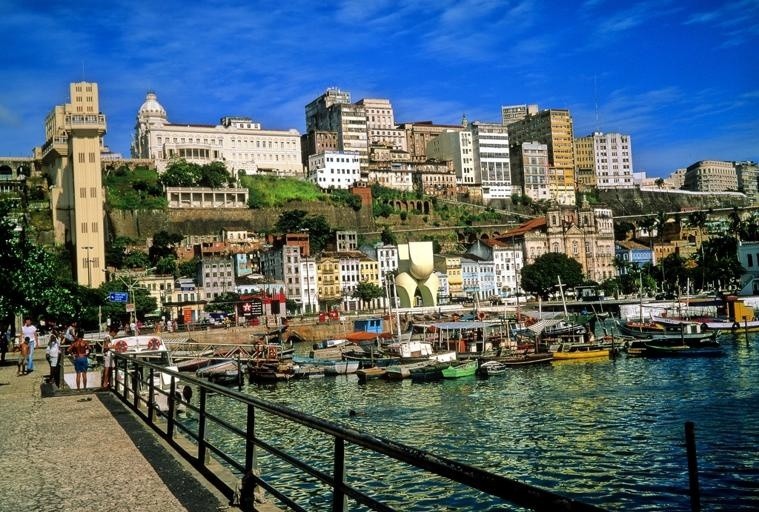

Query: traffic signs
[110,292,128,303]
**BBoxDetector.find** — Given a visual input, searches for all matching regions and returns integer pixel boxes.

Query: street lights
[101,267,157,322]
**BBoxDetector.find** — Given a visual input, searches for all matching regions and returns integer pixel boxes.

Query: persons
[16,316,77,387]
[66,330,91,392]
[100,325,119,390]
[0,333,9,362]
[120,313,232,336]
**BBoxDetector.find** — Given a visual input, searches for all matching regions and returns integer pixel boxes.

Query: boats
[410,360,460,381]
[107,335,186,413]
[354,366,388,381]
[610,274,759,356]
[173,339,359,384]
[441,358,479,378]
[384,350,457,379]
[480,360,507,377]
[332,275,626,360]
